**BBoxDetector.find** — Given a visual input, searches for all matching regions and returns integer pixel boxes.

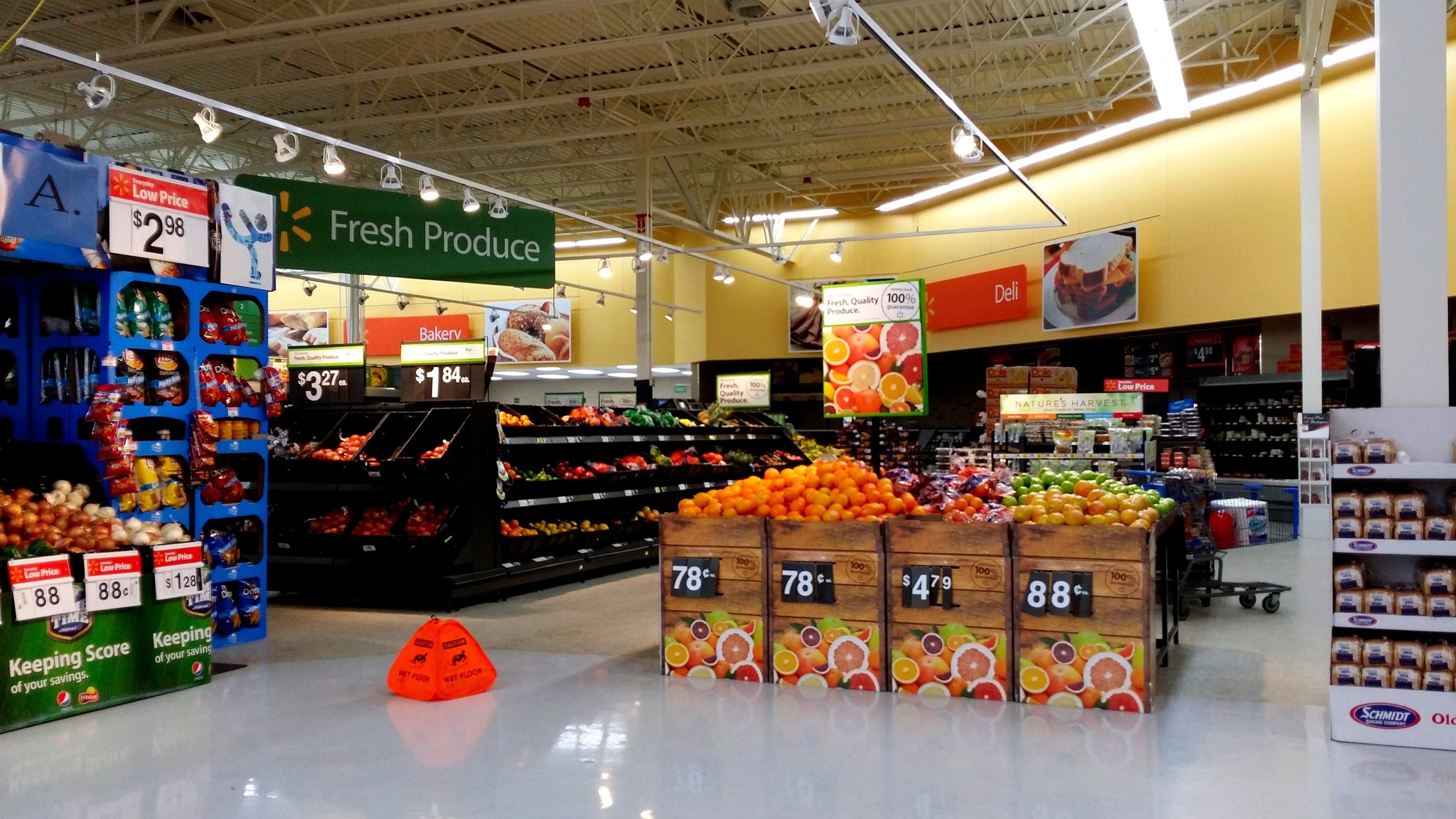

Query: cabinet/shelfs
[0,263,269,649]
[1327,408,1456,751]
[268,481,472,566]
[1198,371,1352,538]
[499,433,782,589]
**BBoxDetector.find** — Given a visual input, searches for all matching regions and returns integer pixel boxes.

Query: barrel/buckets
[1209,511,1235,549]
[1185,528,1200,539]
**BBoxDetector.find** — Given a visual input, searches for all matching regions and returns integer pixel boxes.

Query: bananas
[792,432,855,460]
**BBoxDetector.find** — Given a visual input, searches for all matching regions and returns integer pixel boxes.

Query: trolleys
[1159,473,1301,622]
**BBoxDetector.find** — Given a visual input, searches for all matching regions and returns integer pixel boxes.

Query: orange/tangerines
[773,618,880,691]
[678,456,1175,529]
[664,610,763,683]
[1020,632,1144,713]
[822,321,924,415]
[499,409,533,426]
[501,519,538,535]
[503,462,522,478]
[891,621,1008,701]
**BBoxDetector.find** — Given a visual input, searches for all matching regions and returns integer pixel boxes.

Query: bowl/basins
[1158,407,1214,477]
[936,448,989,474]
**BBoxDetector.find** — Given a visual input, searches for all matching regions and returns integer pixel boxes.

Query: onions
[0,480,191,554]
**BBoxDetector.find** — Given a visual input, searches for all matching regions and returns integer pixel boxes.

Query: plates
[1043,250,1137,328]
[789,306,823,350]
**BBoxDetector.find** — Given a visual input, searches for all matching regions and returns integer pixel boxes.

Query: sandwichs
[1055,232,1135,316]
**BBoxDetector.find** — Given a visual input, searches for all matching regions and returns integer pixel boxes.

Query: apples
[308,433,450,535]
[513,461,594,480]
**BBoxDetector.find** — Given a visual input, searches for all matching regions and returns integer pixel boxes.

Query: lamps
[557,240,675,321]
[77,71,117,111]
[302,279,500,322]
[809,0,861,46]
[713,264,734,285]
[830,242,844,262]
[379,162,508,218]
[273,131,299,162]
[193,104,223,143]
[323,145,345,174]
[951,118,984,163]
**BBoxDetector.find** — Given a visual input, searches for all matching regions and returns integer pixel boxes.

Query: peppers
[0,539,56,560]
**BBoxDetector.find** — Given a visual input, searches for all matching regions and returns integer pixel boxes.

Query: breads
[1332,436,1456,691]
[498,302,569,361]
[266,312,327,356]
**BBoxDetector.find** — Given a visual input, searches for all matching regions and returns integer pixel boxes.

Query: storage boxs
[497,404,804,562]
[268,406,474,556]
[1277,330,1352,373]
[986,365,1077,446]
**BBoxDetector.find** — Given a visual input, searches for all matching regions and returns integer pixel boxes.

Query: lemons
[529,506,659,534]
